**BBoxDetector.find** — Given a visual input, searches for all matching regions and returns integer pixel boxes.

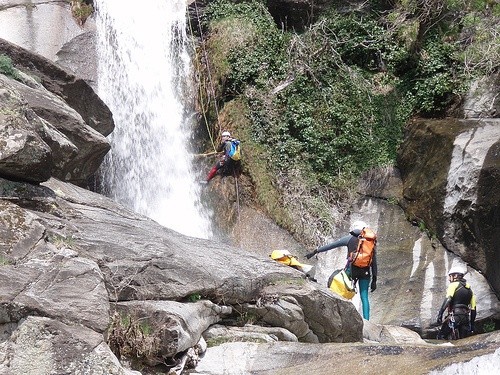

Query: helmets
[222,132,230,136]
[448,264,465,275]
[349,221,368,230]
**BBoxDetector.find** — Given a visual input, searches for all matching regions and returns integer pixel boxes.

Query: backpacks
[348,227,377,268]
[225,140,242,161]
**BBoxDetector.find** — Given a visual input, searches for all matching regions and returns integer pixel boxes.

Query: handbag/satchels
[327,267,357,300]
[268,249,302,270]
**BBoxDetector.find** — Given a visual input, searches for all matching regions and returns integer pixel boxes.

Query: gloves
[468,321,474,332]
[304,249,318,259]
[371,279,377,292]
[437,311,442,323]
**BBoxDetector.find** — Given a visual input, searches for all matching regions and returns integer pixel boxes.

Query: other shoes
[199,181,208,185]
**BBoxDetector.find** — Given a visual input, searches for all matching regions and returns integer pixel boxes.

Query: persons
[303,222,377,320]
[436,264,476,341]
[199,131,237,185]
